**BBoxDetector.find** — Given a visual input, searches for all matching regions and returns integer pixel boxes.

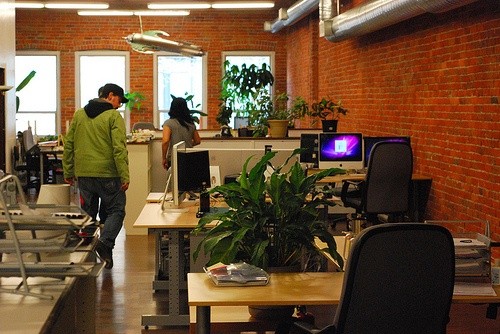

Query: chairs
[17,131,58,181]
[340,139,413,225]
[14,145,38,192]
[293,221,456,334]
[22,129,62,183]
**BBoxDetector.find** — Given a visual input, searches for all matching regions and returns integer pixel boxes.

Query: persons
[62,83,129,269]
[161,97,200,169]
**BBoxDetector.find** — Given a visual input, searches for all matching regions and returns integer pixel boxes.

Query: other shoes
[95,239,113,269]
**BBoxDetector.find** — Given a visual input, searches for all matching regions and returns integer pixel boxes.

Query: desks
[0,146,500,334]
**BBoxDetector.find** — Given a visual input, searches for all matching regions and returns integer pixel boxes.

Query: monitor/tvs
[318,132,364,169]
[161,141,189,213]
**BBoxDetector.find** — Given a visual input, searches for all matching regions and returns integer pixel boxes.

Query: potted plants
[267,91,305,138]
[312,95,349,133]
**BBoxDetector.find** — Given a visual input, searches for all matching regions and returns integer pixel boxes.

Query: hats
[99,84,129,103]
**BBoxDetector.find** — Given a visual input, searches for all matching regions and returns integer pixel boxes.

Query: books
[455,250,482,276]
